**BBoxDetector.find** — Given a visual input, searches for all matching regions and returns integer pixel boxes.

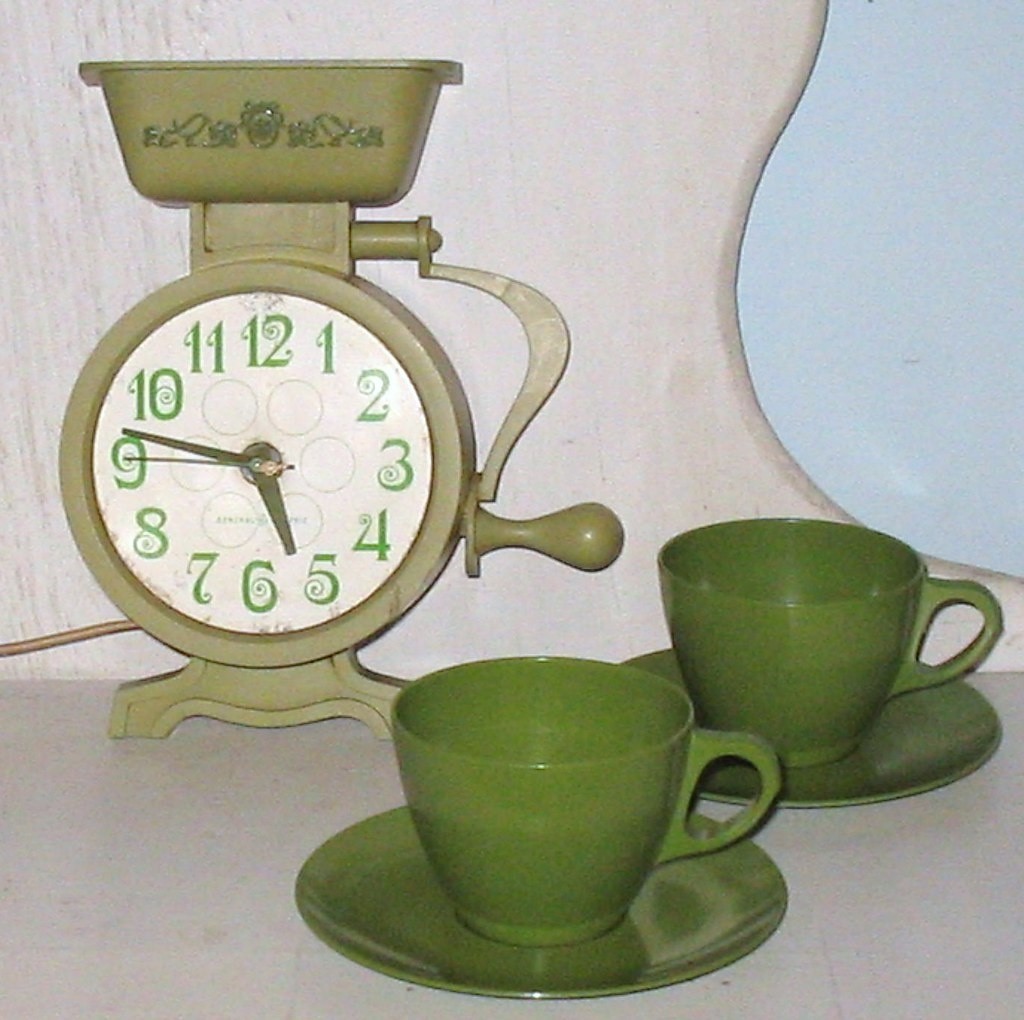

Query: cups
[655,519,1005,772]
[388,655,785,949]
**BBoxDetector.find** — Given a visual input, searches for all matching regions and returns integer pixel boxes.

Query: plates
[619,648,1004,811]
[296,804,792,1000]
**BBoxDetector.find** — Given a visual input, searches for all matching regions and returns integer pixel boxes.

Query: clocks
[56,60,623,742]
